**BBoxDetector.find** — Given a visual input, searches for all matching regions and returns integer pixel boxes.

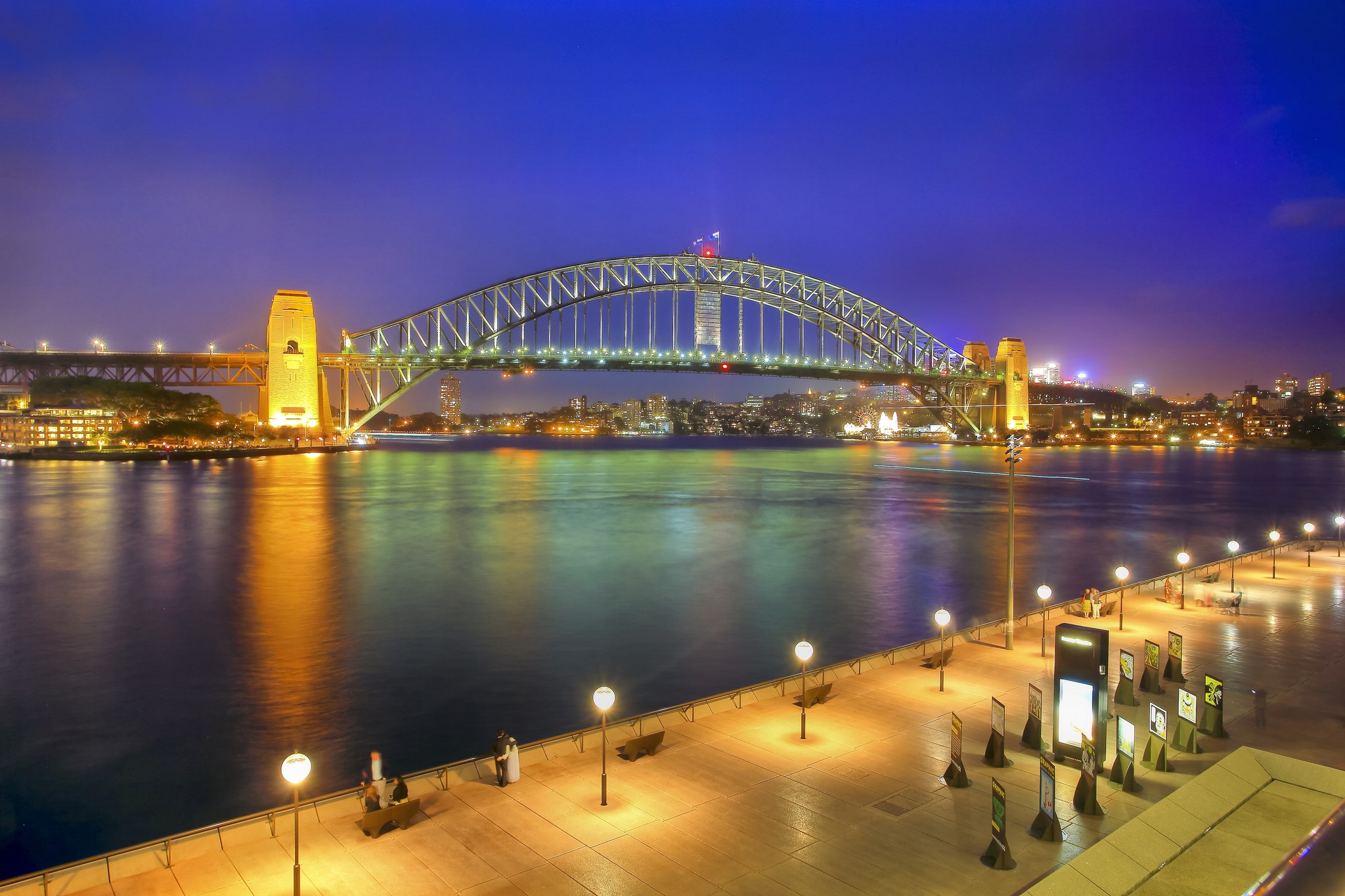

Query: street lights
[934,607,951,692]
[1268,531,1280,579]
[1005,434,1024,651]
[1227,540,1240,592]
[593,685,616,806]
[1334,516,1345,556]
[1036,583,1053,657]
[1176,552,1190,610]
[281,750,312,896]
[1115,566,1130,630]
[1303,522,1315,567]
[794,638,814,739]
